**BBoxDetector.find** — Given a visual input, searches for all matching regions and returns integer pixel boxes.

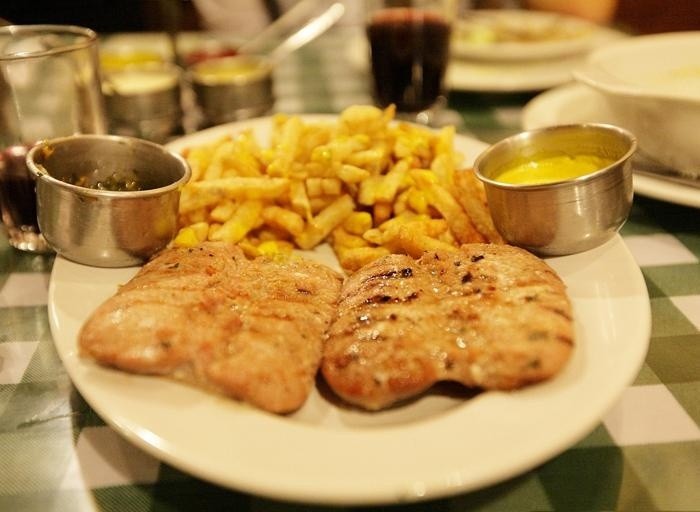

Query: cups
[0,26,100,253]
[364,1,453,121]
[21,131,193,270]
[471,120,639,259]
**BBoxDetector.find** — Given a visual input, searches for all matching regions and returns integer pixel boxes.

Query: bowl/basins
[573,31,700,182]
[98,54,273,144]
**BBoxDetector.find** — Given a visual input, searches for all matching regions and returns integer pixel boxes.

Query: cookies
[322,242,573,409]
[78,244,344,414]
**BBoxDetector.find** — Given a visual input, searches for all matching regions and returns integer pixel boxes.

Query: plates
[517,83,700,209]
[352,10,627,95]
[47,114,652,508]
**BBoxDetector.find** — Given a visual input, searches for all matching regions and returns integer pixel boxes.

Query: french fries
[177,103,502,272]
